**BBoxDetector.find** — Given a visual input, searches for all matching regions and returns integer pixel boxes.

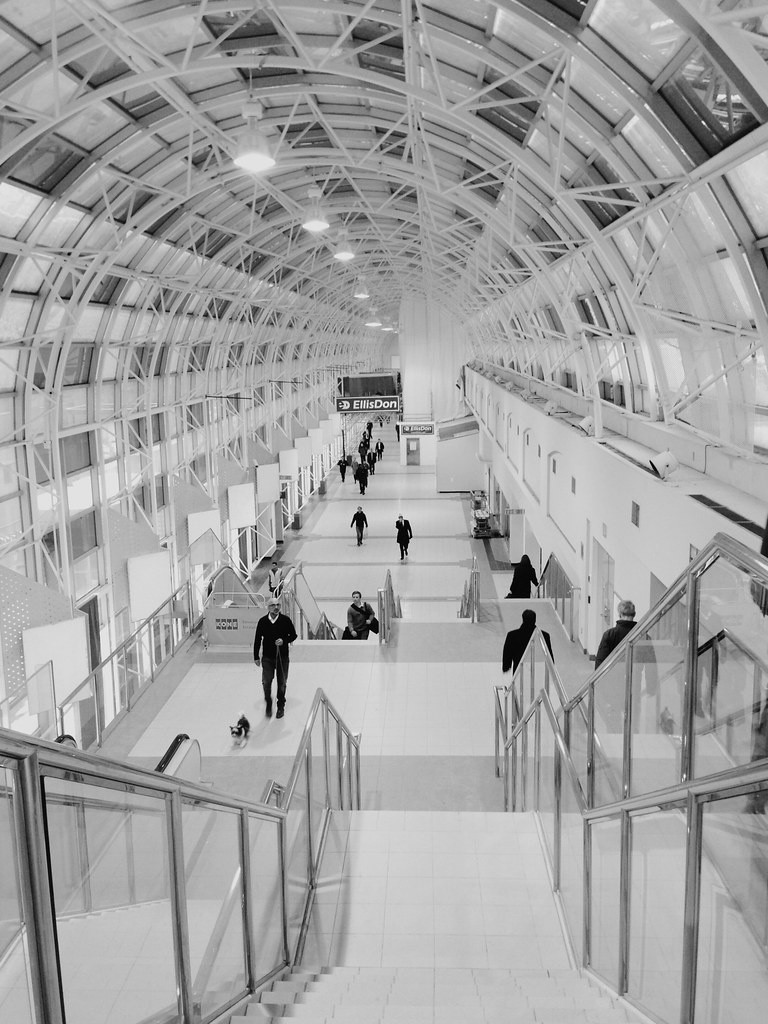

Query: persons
[351,506,368,546]
[347,591,375,639]
[351,415,399,496]
[396,516,413,560]
[268,562,284,598]
[510,555,539,598]
[337,456,350,482]
[503,609,555,678]
[254,597,298,718]
[594,600,658,736]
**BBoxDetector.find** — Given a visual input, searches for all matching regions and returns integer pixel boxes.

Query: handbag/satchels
[368,617,379,634]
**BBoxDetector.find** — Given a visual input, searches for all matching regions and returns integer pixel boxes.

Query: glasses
[268,603,281,607]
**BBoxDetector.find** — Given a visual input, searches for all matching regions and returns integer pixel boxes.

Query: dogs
[229,713,250,747]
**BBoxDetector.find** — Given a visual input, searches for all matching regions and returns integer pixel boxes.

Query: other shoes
[276,706,284,718]
[266,702,272,717]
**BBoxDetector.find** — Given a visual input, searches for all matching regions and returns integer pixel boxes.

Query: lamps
[234,102,275,169]
[649,450,678,479]
[366,308,381,327]
[355,279,369,299]
[519,388,532,401]
[543,400,557,413]
[335,227,353,259]
[381,315,393,331]
[579,415,595,436]
[466,360,515,390]
[300,185,329,231]
